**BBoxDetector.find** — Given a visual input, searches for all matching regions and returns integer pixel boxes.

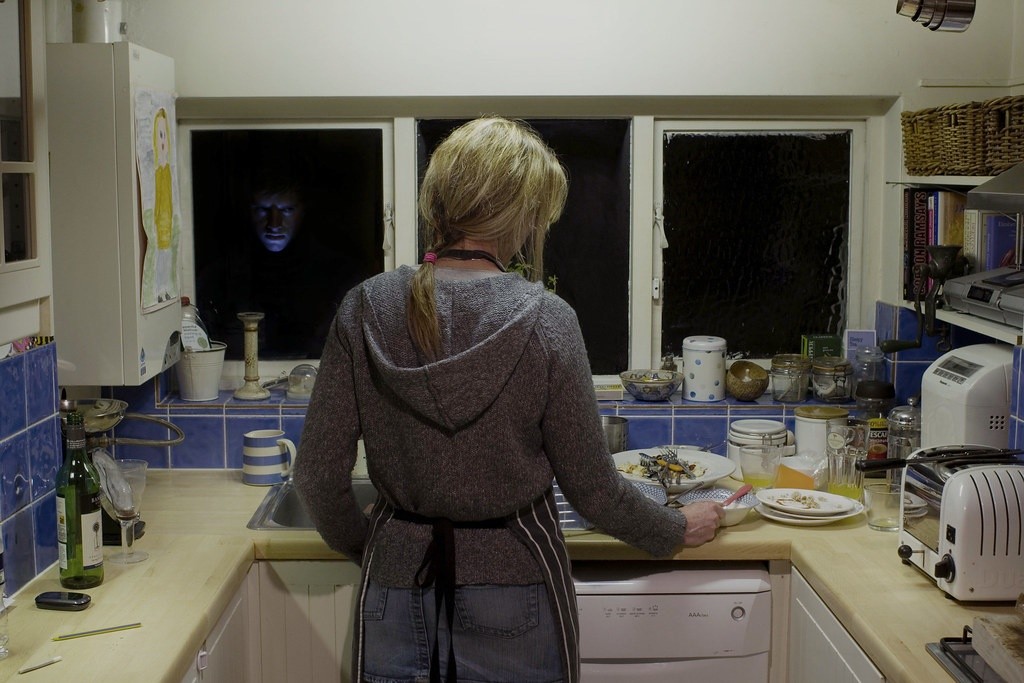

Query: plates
[759,497,864,519]
[755,488,853,515]
[627,480,668,505]
[652,445,711,454]
[608,450,736,494]
[754,505,843,526]
[904,489,927,519]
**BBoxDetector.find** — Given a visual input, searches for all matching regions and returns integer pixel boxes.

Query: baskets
[899,105,943,176]
[936,101,988,176]
[981,95,1023,176]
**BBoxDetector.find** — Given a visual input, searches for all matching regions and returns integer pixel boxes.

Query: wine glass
[101,459,149,564]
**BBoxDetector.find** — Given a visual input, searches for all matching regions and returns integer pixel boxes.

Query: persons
[296,111,730,683]
[242,165,325,361]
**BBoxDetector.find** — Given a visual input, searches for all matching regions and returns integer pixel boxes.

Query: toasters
[899,444,1024,602]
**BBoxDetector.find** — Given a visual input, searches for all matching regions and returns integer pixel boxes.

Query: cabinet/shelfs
[180,566,259,681]
[779,567,887,683]
[888,175,1024,352]
[250,558,360,683]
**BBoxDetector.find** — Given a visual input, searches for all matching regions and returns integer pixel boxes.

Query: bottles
[179,296,213,351]
[0,551,9,659]
[54,411,104,588]
[812,356,851,403]
[661,353,677,372]
[886,397,921,493]
[853,381,893,462]
[772,354,811,403]
[850,346,886,401]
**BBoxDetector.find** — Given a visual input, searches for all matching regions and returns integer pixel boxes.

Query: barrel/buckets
[599,415,629,453]
[175,342,228,402]
[682,336,727,401]
[793,404,851,457]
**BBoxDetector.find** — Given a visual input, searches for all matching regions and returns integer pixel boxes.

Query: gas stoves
[923,617,1024,683]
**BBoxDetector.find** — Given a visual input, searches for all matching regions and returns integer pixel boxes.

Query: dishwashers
[567,564,772,683]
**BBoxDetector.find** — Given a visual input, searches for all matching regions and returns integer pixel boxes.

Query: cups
[739,445,781,494]
[888,431,918,489]
[826,419,869,500]
[864,484,899,529]
[242,428,298,487]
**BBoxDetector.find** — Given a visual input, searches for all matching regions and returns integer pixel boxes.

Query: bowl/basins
[727,360,769,401]
[619,368,685,401]
[676,488,761,527]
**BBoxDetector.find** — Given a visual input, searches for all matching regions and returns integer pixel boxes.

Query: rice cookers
[728,417,795,482]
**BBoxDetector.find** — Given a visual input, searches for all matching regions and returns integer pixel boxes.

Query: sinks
[246,480,379,537]
